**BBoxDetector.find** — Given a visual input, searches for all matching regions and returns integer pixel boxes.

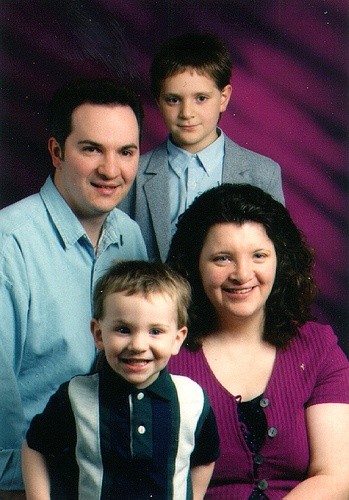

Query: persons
[118,30,288,264]
[2,79,148,494]
[164,182,348,499]
[19,261,220,499]
